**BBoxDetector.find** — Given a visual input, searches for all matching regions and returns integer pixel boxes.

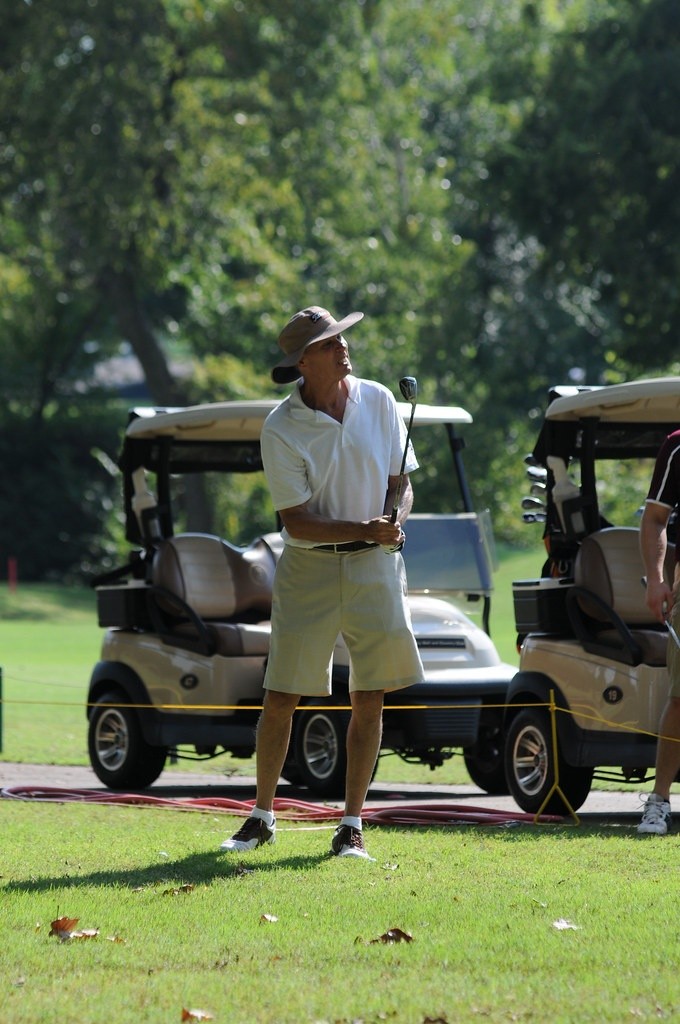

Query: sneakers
[331,824,369,859]
[636,792,671,833]
[220,817,276,852]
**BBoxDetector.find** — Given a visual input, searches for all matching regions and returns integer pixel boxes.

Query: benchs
[577,527,670,670]
[153,531,285,658]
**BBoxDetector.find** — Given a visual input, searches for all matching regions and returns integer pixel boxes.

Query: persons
[638,431,680,836]
[216,305,419,858]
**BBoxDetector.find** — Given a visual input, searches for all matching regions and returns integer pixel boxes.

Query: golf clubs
[388,377,417,524]
[642,575,680,647]
[520,453,549,524]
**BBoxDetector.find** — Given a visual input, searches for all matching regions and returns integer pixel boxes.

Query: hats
[271,305,364,384]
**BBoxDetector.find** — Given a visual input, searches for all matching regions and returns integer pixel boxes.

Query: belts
[313,541,380,554]
